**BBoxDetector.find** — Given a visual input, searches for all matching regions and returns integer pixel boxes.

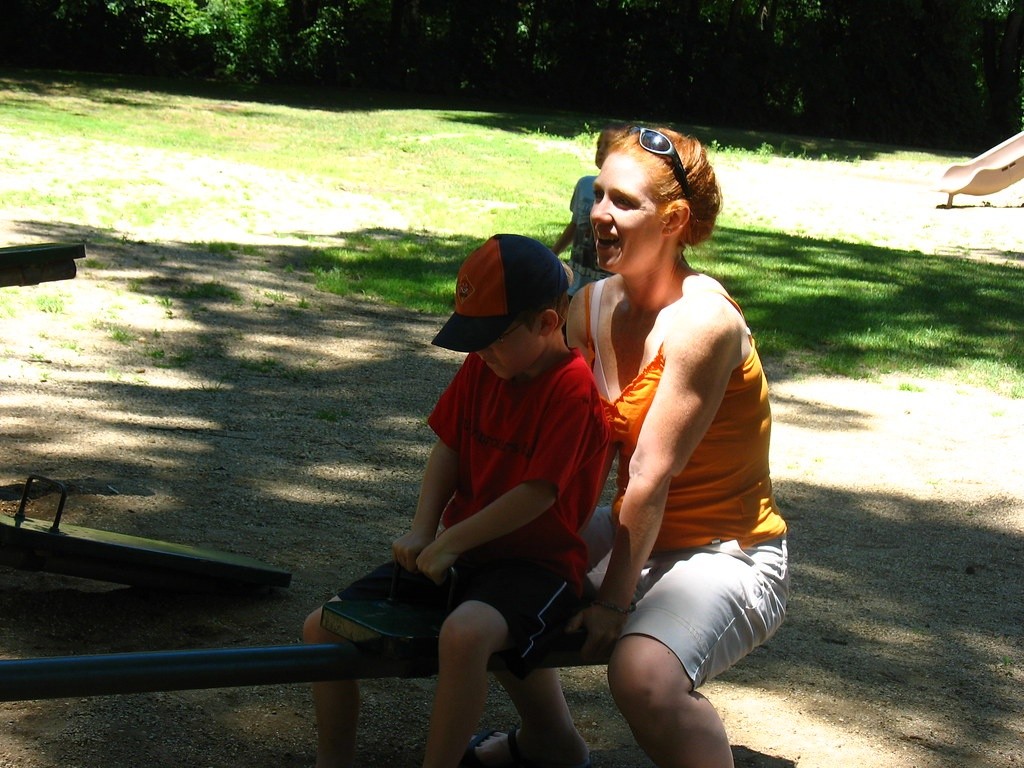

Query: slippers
[461,729,592,768]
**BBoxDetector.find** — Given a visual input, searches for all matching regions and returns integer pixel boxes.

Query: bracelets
[593,601,636,614]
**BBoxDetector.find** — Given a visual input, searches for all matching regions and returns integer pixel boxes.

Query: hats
[431,234,569,352]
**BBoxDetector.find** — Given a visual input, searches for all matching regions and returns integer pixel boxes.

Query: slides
[930,130,1024,196]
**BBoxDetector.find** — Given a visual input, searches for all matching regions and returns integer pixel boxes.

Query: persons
[466,127,787,768]
[302,234,610,768]
[551,123,626,299]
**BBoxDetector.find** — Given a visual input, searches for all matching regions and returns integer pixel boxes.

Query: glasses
[495,306,565,345]
[622,124,692,199]
